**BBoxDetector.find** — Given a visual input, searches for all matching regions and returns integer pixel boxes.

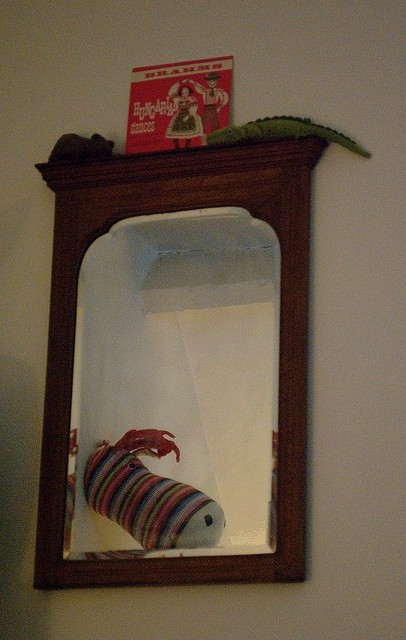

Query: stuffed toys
[204,114,373,163]
[81,427,226,550]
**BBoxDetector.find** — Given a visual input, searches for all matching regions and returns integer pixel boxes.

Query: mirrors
[34,136,330,588]
